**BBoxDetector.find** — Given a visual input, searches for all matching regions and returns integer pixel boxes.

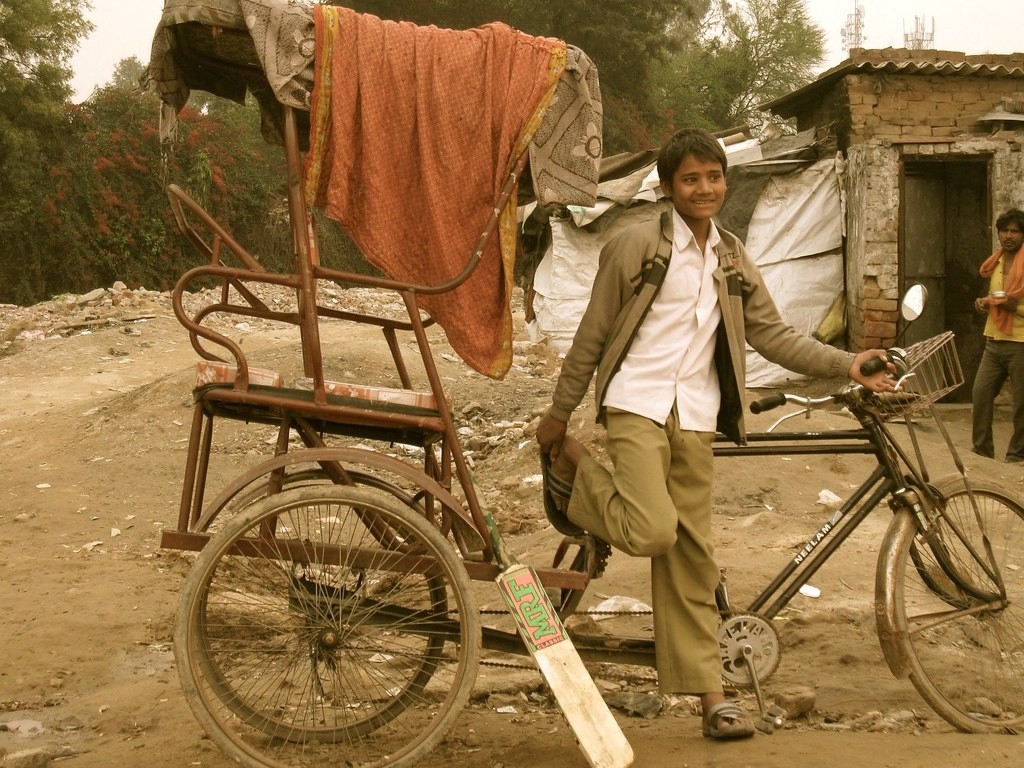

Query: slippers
[538,446,585,536]
[702,703,756,739]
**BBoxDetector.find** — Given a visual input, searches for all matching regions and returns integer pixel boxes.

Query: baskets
[835,331,965,419]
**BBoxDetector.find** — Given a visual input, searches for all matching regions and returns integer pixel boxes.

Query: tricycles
[135,1,1023,767]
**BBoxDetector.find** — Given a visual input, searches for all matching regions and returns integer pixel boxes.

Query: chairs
[163,181,457,553]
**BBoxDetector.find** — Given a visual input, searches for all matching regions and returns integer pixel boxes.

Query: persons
[972,209,1024,463]
[537,127,906,739]
